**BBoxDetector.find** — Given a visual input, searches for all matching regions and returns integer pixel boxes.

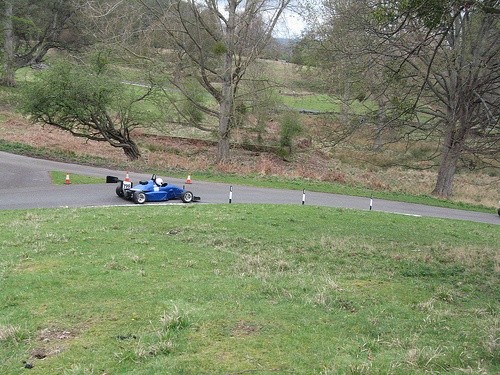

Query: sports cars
[105,174,200,204]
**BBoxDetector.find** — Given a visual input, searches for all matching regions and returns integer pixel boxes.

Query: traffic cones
[124,172,132,183]
[185,174,193,184]
[64,173,71,184]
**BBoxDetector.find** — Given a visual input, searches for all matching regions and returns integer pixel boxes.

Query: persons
[154,178,168,190]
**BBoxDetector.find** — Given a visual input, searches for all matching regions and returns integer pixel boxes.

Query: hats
[156,178,163,185]
